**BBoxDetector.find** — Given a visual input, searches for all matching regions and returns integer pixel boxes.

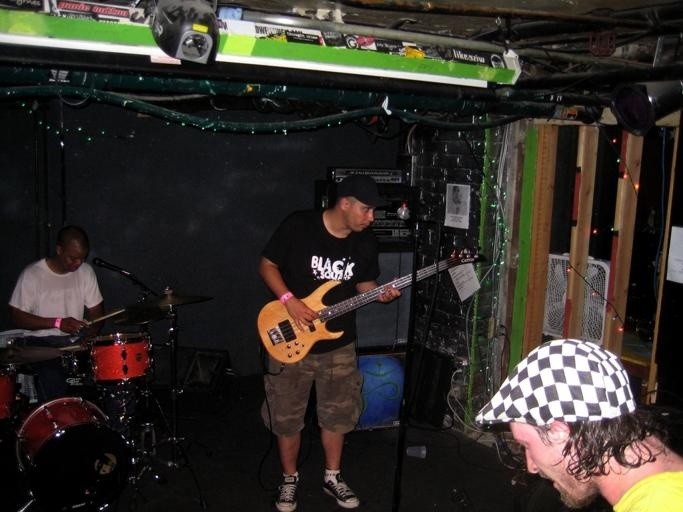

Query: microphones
[93,256,131,276]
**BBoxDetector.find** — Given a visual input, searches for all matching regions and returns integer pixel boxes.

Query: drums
[16,397,126,508]
[1,363,22,425]
[90,332,154,384]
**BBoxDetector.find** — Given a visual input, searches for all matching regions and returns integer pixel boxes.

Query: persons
[8,225,107,403]
[255,175,402,512]
[472,337,683,512]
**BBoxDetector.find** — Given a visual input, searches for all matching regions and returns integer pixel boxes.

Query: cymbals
[1,347,61,366]
[108,308,171,324]
[128,294,215,308]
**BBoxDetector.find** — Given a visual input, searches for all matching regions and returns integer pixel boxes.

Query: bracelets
[54,318,61,329]
[278,292,293,304]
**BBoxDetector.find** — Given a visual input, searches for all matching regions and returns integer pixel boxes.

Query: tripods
[135,309,215,512]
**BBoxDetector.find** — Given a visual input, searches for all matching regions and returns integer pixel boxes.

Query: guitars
[257,245,487,363]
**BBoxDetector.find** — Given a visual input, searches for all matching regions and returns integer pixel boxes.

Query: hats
[473,336,636,428]
[333,173,392,209]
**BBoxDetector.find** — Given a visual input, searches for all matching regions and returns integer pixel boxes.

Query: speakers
[344,241,419,356]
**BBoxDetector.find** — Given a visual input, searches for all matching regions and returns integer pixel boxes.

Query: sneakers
[321,473,360,508]
[274,474,301,512]
